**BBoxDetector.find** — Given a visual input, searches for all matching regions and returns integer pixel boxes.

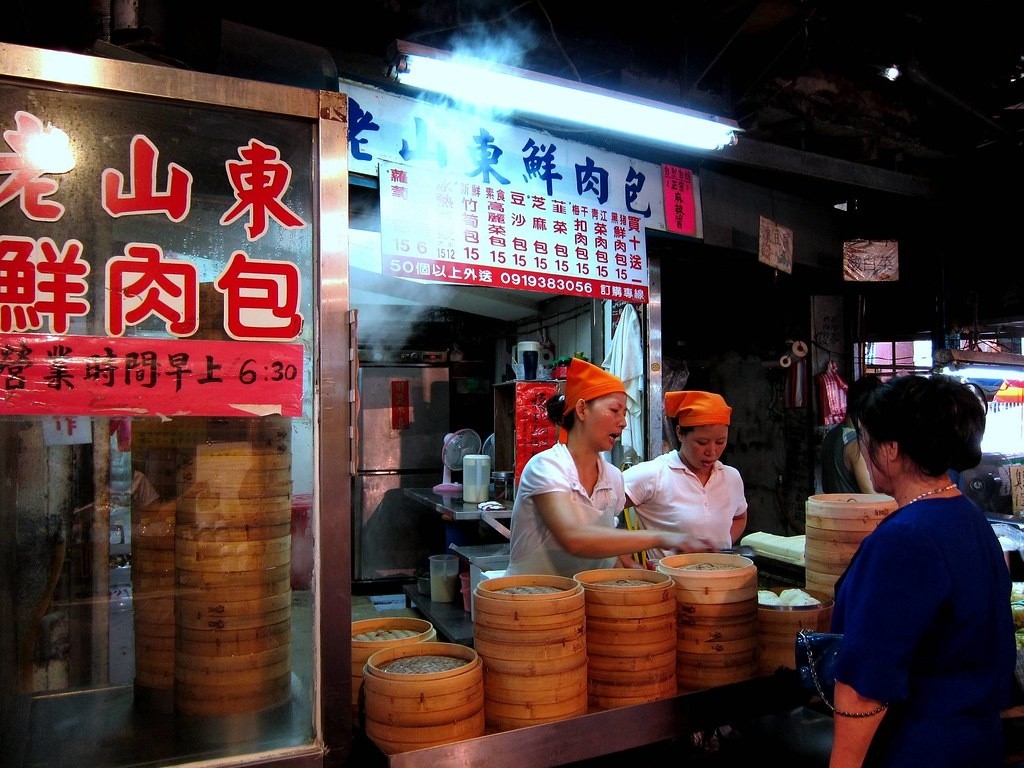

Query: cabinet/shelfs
[401,487,515,645]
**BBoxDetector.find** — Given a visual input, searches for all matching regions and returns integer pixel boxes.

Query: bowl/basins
[412,570,431,594]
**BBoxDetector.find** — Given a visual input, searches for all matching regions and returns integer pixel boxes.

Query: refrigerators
[346,347,450,590]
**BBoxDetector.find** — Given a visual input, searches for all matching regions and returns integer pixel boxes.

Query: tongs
[689,545,755,558]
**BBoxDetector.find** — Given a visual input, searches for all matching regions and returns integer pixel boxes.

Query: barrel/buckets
[290,495,314,589]
[460,573,472,610]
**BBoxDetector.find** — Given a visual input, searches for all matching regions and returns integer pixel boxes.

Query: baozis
[758,588,820,607]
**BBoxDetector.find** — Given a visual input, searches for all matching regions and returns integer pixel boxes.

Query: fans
[433,428,482,499]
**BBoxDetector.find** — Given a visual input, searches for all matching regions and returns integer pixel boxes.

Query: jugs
[511,342,543,381]
[463,454,491,502]
[428,554,459,601]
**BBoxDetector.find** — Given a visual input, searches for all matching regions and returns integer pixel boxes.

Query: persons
[131,459,160,525]
[507,358,707,577]
[621,391,748,569]
[830,375,1016,768]
[822,374,884,493]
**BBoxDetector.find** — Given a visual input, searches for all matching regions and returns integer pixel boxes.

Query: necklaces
[908,483,957,504]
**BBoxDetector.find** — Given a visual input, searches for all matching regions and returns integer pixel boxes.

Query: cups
[523,350,538,379]
[514,364,525,379]
